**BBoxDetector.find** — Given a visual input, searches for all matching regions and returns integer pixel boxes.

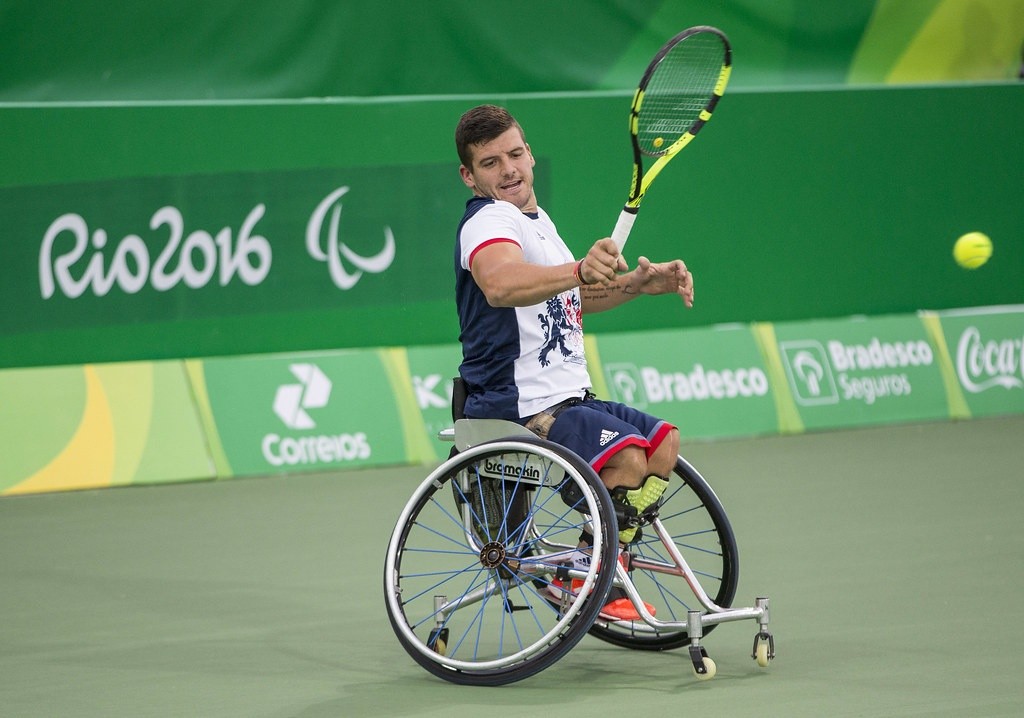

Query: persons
[455,105,695,621]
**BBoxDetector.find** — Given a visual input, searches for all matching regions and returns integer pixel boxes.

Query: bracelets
[573,261,582,285]
[577,258,587,284]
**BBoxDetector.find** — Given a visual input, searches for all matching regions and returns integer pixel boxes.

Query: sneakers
[549,550,656,621]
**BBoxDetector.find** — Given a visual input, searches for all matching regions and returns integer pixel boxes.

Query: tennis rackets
[608,25,735,261]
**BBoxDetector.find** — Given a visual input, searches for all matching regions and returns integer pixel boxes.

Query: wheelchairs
[379,374,775,690]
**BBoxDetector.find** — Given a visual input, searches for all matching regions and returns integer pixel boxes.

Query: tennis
[953,230,995,270]
